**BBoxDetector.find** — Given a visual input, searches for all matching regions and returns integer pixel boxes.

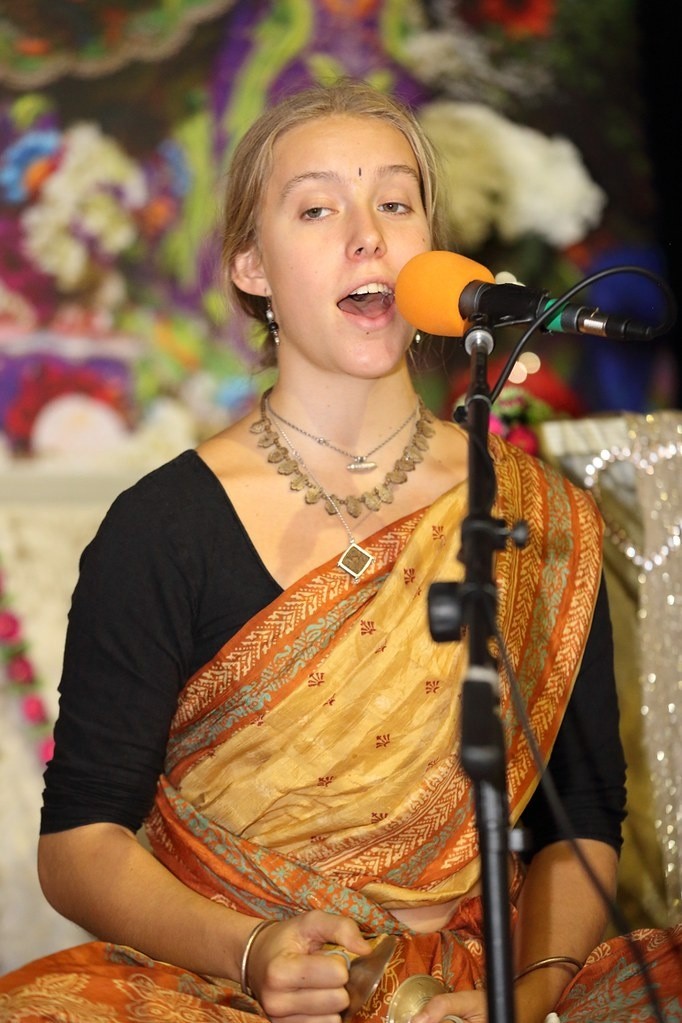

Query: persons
[0,70,681,1022]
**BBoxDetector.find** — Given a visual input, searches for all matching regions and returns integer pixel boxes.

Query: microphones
[395,251,655,341]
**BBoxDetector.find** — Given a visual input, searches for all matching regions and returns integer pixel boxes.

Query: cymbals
[343,935,397,1018]
[385,973,449,1023]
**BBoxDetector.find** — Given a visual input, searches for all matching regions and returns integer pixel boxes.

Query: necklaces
[250,385,435,581]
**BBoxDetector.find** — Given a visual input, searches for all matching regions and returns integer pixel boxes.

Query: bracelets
[238,917,283,995]
[515,956,585,980]
